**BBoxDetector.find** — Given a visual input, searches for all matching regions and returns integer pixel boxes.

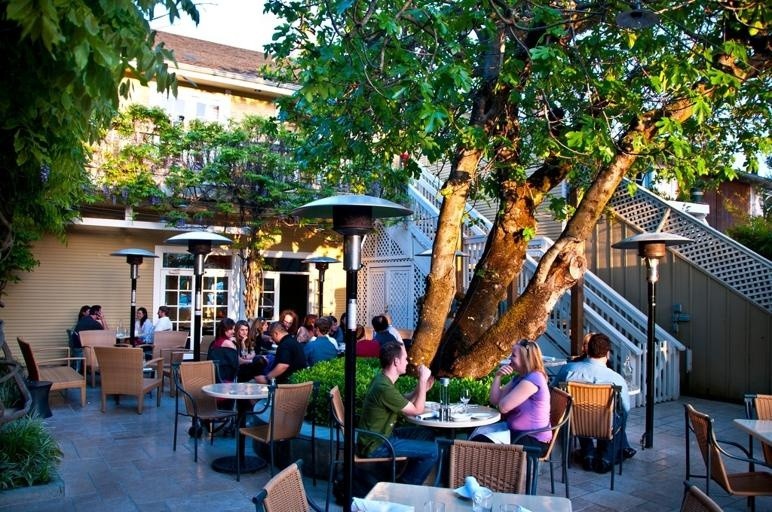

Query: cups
[500,503,523,511]
[115,325,128,336]
[423,500,445,512]
[241,348,248,360]
[473,487,494,511]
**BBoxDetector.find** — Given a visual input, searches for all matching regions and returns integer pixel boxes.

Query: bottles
[621,359,633,388]
[270,375,277,385]
[250,347,255,353]
[439,404,444,421]
[440,377,450,405]
[446,404,451,420]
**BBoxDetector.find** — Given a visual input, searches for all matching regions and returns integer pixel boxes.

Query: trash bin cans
[26,381,53,419]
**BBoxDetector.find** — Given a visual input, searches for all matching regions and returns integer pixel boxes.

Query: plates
[471,412,493,420]
[456,485,493,499]
[420,410,437,420]
[450,413,473,421]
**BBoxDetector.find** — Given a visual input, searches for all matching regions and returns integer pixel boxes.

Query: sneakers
[583,458,611,474]
[624,448,637,459]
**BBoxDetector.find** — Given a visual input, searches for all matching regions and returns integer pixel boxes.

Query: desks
[498,354,567,380]
[403,400,503,487]
[349,479,574,512]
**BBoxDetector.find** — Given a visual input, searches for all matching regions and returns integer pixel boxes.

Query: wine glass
[460,388,471,413]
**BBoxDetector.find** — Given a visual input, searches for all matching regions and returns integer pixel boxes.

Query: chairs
[557,379,624,498]
[328,385,407,504]
[432,436,545,495]
[245,457,333,512]
[512,385,575,494]
[682,390,772,512]
[15,335,88,411]
[67,329,412,483]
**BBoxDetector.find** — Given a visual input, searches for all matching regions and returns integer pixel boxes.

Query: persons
[489,336,554,457]
[134,306,155,347]
[549,333,632,473]
[569,332,637,465]
[354,339,440,485]
[206,307,406,427]
[73,304,90,331]
[69,304,109,374]
[142,305,173,344]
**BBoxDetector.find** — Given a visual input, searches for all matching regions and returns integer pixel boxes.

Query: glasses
[521,339,529,358]
